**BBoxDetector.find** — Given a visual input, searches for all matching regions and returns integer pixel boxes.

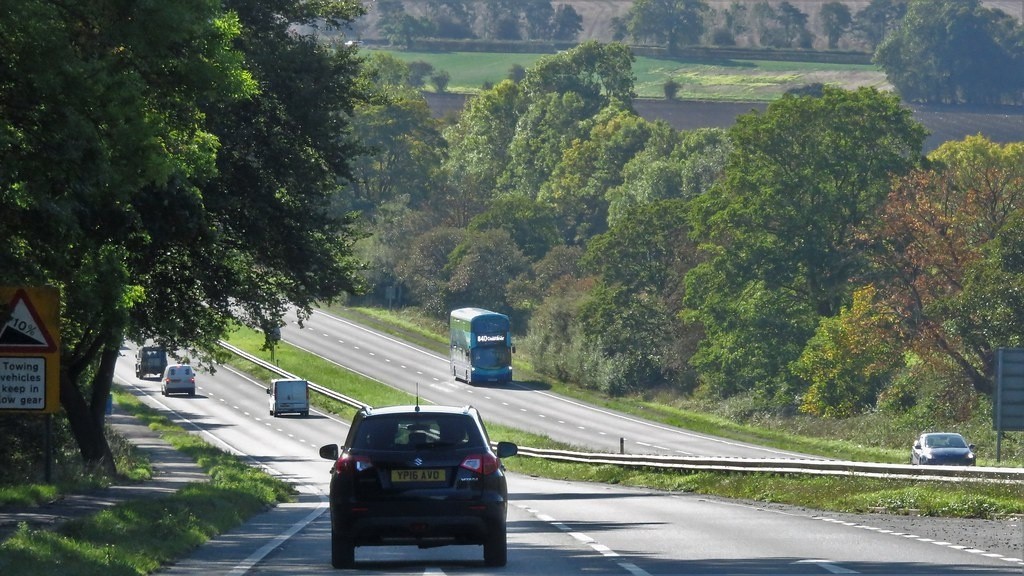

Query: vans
[160,364,196,397]
[266,378,311,417]
[134,345,168,379]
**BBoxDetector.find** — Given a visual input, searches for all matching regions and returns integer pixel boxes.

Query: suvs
[319,405,519,568]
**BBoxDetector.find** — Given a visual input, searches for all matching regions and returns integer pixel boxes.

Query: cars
[910,432,977,467]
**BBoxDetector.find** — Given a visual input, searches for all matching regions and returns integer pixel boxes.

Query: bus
[450,307,516,385]
